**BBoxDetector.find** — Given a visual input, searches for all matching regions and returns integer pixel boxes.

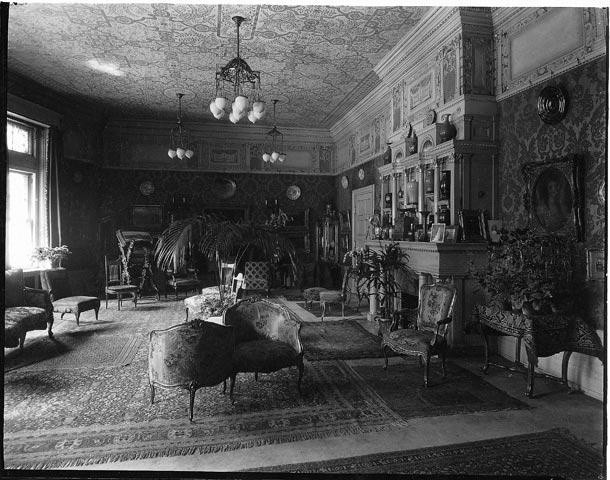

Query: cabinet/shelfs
[376,140,498,244]
[315,215,339,264]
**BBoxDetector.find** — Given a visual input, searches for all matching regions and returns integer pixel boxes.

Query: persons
[534,175,571,231]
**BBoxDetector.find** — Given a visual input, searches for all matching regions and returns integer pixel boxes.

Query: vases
[439,113,455,141]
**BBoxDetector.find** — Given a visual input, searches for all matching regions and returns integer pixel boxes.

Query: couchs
[5,268,53,349]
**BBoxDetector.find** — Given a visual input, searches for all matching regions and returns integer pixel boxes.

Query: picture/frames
[519,152,586,242]
[585,247,605,282]
[339,210,352,232]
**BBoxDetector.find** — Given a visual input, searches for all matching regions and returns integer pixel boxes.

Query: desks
[463,303,603,399]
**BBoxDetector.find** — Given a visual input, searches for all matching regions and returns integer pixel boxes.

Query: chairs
[380,282,458,391]
[201,259,240,296]
[229,295,304,396]
[162,254,200,297]
[41,267,100,328]
[184,258,223,321]
[103,254,138,311]
[146,317,243,421]
[232,272,249,301]
[320,267,354,323]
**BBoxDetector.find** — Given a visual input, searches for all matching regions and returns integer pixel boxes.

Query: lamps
[166,15,286,166]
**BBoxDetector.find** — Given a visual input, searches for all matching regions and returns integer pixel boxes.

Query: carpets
[3,280,534,471]
[232,426,605,480]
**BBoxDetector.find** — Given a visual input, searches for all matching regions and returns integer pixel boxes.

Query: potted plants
[348,241,420,338]
